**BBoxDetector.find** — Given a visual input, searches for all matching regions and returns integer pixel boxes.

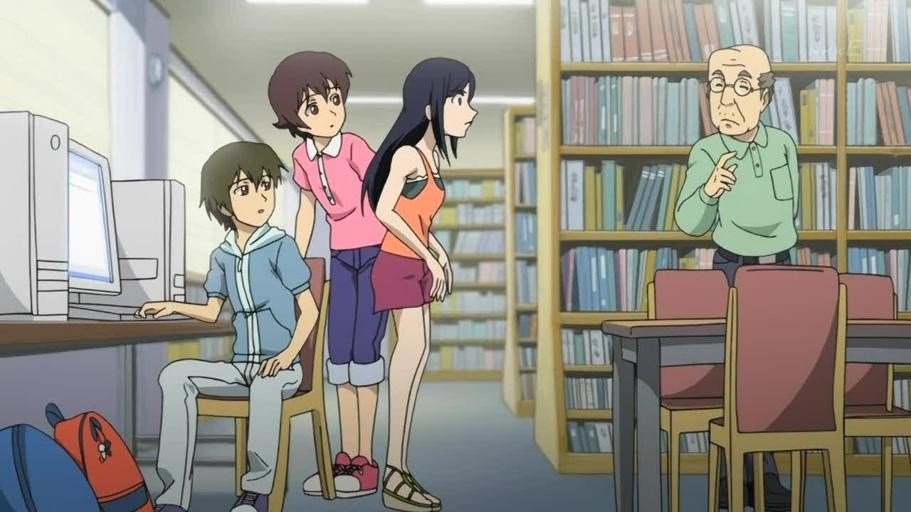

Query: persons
[357,55,483,511]
[133,140,321,511]
[671,43,807,510]
[267,49,392,500]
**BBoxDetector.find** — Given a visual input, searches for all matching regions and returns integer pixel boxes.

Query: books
[556,0,911,454]
[405,114,538,406]
